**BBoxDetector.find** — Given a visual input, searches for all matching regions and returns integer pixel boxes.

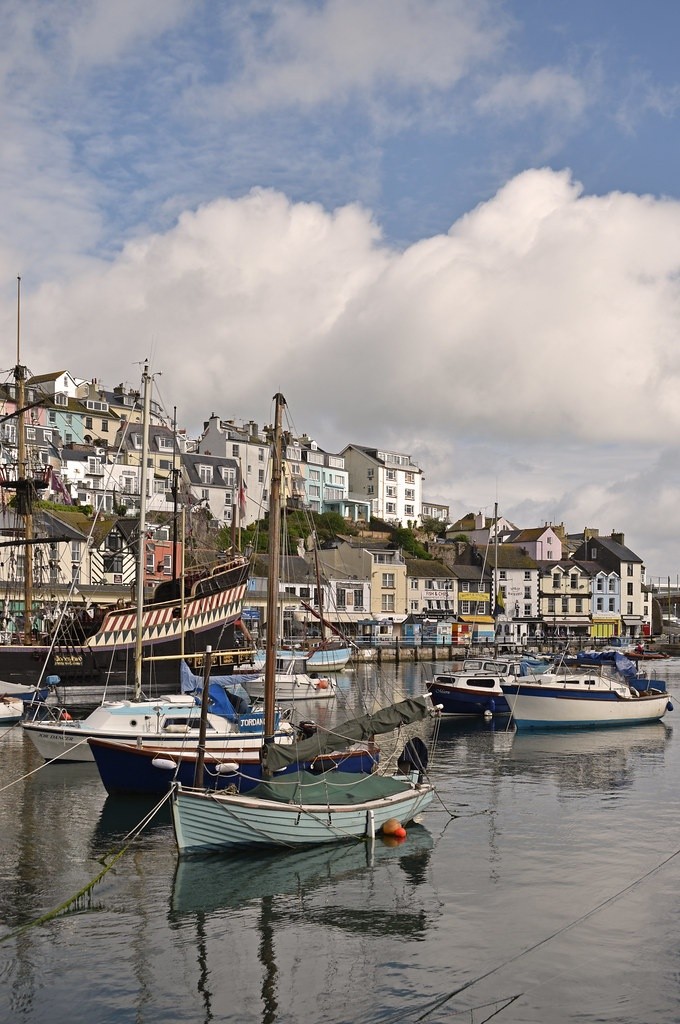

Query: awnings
[623,619,644,626]
[545,620,594,626]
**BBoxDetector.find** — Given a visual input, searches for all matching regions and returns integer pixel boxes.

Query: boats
[426,652,559,718]
[501,651,673,733]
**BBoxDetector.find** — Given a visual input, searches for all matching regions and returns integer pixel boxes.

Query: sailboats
[21,360,296,762]
[1,273,252,722]
[167,389,438,857]
